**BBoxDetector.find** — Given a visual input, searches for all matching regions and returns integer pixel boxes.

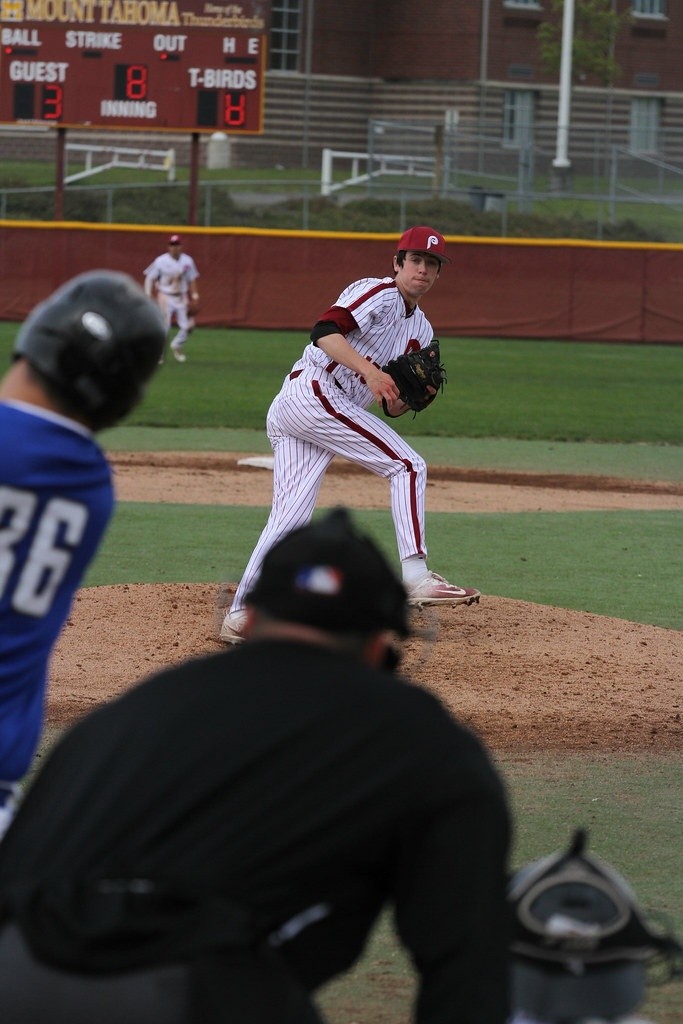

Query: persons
[143,235,201,363]
[222,226,481,645]
[0,502,521,1024]
[0,271,163,839]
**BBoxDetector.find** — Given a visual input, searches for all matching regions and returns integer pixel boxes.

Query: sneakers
[402,570,480,612]
[220,614,247,647]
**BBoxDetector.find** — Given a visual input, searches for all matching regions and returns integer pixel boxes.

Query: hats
[396,226,452,266]
[167,236,182,244]
[245,505,409,640]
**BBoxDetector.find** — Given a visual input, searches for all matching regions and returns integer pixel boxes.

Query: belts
[0,787,10,809]
[334,379,342,389]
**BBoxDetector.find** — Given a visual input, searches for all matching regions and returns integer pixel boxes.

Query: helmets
[10,269,166,433]
[500,825,683,1024]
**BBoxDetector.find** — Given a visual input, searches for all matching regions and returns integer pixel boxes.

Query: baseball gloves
[380,338,449,423]
[184,299,202,320]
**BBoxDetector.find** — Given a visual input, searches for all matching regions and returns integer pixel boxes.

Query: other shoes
[172,343,185,362]
